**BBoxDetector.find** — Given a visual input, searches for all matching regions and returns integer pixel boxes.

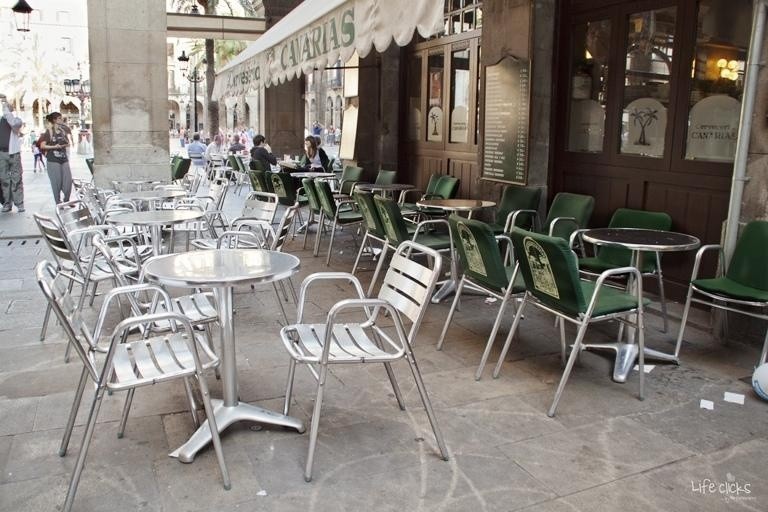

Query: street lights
[9,2,33,32]
[62,79,72,96]
[71,80,81,97]
[188,5,200,15]
[177,51,188,72]
[202,58,211,77]
[82,80,90,98]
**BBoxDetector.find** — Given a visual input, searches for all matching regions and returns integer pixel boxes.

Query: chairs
[74,178,136,231]
[504,192,596,267]
[217,201,301,309]
[436,213,528,379]
[236,157,250,197]
[672,220,768,368]
[92,232,239,396]
[322,165,364,195]
[156,170,202,209]
[212,192,279,248]
[347,190,437,285]
[280,239,445,483]
[162,178,229,248]
[397,175,455,222]
[55,199,140,305]
[371,170,398,198]
[366,195,464,317]
[314,178,376,267]
[553,207,673,333]
[492,225,646,418]
[35,258,232,511]
[402,177,461,230]
[467,185,543,234]
[228,156,249,186]
[301,177,354,250]
[32,212,140,364]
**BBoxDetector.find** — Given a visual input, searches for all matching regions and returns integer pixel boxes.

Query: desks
[115,178,154,191]
[106,210,207,256]
[355,182,416,198]
[289,172,336,178]
[107,190,187,211]
[583,226,702,339]
[415,198,497,220]
[142,248,307,464]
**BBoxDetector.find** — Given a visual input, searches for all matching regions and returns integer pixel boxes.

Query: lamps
[717,58,739,84]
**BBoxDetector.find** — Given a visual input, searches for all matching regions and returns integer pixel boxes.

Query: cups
[283,154,290,163]
[295,156,299,162]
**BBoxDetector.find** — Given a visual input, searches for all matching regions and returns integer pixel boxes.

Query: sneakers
[1,203,9,212]
[18,204,24,210]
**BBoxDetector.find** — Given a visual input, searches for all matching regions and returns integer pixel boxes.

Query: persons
[304,135,332,172]
[250,135,278,174]
[227,134,245,155]
[180,127,189,147]
[328,125,335,147]
[313,120,324,137]
[188,133,207,165]
[206,135,221,153]
[31,141,46,173]
[40,112,76,211]
[335,126,340,137]
[0,95,27,213]
[30,131,37,143]
